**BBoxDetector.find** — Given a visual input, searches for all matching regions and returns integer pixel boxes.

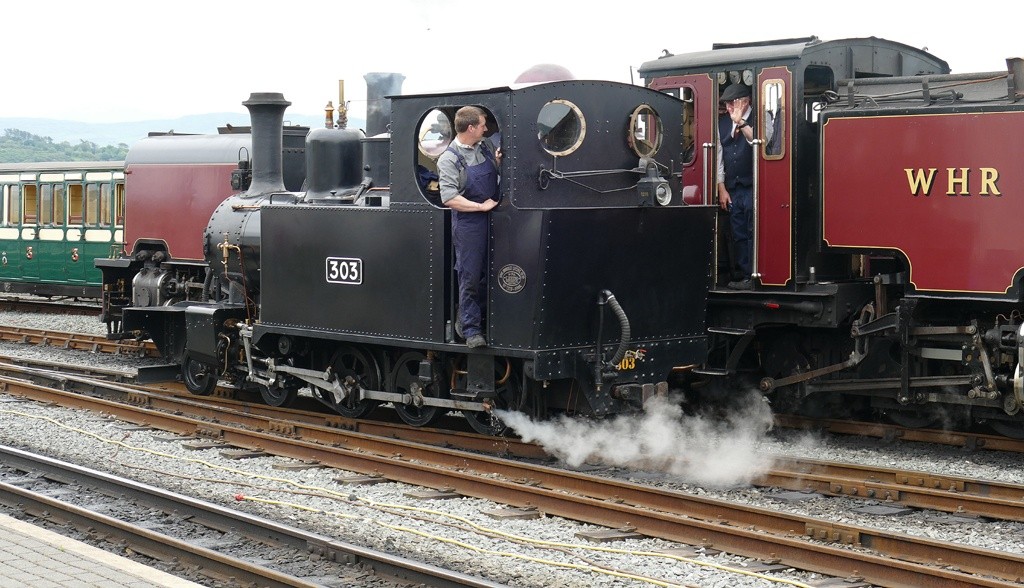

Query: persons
[436,104,502,349]
[716,83,774,289]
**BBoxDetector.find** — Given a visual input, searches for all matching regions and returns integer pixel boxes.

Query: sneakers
[728,279,753,290]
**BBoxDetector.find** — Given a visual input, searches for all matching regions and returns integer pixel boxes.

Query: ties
[733,124,740,141]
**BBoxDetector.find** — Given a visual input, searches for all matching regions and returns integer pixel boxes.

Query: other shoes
[467,334,486,347]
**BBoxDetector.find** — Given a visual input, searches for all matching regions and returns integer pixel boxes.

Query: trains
[92,38,1024,448]
[0,159,126,305]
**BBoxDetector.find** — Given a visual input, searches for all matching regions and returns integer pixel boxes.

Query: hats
[720,83,751,101]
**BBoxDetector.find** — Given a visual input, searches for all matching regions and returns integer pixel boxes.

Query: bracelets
[738,120,748,129]
